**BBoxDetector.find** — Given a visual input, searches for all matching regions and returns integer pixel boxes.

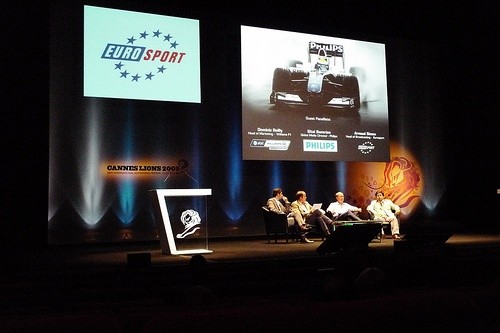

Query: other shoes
[332,222,342,225]
[301,226,312,232]
[392,234,400,239]
[301,238,314,243]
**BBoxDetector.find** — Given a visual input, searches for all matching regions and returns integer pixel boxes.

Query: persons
[267,188,314,242]
[366,190,402,239]
[292,191,333,239]
[326,192,362,221]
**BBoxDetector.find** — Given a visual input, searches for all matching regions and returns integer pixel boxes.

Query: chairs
[262,206,401,244]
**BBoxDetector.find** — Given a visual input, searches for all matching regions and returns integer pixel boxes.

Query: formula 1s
[268,40,366,125]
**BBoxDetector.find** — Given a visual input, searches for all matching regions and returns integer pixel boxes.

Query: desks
[331,220,389,242]
